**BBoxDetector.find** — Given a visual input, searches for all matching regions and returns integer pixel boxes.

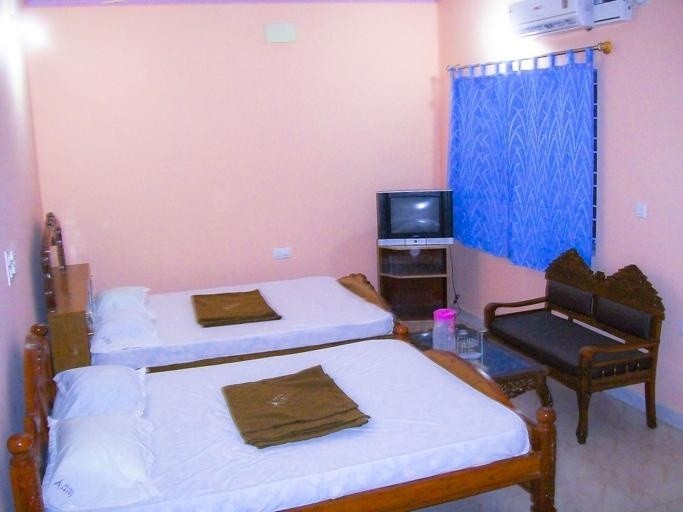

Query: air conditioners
[505,0,633,39]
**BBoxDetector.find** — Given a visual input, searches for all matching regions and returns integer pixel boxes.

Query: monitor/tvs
[376,189,455,245]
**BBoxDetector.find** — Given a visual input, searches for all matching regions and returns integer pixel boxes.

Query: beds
[38,215,410,373]
[10,322,558,508]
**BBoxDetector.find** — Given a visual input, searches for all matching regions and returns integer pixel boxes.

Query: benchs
[482,249,668,444]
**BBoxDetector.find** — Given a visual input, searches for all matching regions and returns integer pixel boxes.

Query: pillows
[44,416,157,504]
[90,308,163,354]
[53,364,151,423]
[94,286,151,310]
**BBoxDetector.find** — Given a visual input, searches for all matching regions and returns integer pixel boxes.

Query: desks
[376,243,449,321]
[406,324,552,407]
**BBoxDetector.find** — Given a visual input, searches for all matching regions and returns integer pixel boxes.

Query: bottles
[432,309,456,354]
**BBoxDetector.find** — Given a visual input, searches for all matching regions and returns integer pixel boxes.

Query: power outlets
[272,247,292,261]
